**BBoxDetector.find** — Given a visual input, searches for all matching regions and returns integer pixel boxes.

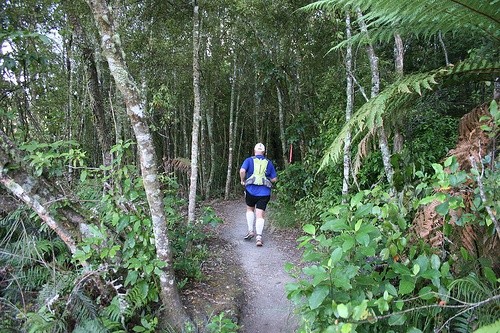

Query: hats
[254,142,265,151]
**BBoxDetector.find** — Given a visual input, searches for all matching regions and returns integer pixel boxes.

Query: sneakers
[244,231,255,240]
[256,236,263,246]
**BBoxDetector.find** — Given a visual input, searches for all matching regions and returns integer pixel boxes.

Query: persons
[239,141,277,246]
